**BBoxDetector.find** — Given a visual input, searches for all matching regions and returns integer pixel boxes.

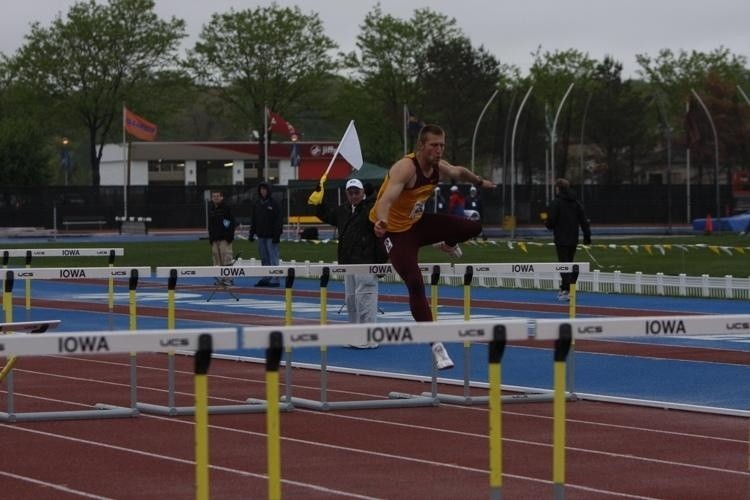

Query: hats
[435,182,476,191]
[346,177,364,192]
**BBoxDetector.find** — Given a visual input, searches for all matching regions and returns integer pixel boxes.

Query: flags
[338,120,364,171]
[123,107,157,142]
[266,108,296,141]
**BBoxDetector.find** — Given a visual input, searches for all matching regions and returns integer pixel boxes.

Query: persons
[248,182,283,288]
[449,186,465,214]
[207,191,236,285]
[434,187,445,215]
[313,179,386,349]
[545,179,591,301]
[465,186,483,222]
[369,124,496,372]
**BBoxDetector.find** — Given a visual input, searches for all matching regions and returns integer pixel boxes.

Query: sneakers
[436,240,464,260]
[430,340,454,370]
[349,340,379,351]
[554,291,571,302]
[212,277,282,289]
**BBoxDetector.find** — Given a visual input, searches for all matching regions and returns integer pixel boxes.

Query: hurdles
[242,315,531,499]
[2,326,238,500]
[535,313,750,499]
[278,262,453,413]
[137,265,309,417]
[421,262,590,405]
[1,266,153,421]
[0,248,125,332]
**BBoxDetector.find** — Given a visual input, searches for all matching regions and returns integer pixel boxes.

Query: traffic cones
[706,214,713,234]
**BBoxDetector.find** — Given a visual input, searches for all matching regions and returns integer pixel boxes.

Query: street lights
[290,131,299,179]
[62,137,70,186]
